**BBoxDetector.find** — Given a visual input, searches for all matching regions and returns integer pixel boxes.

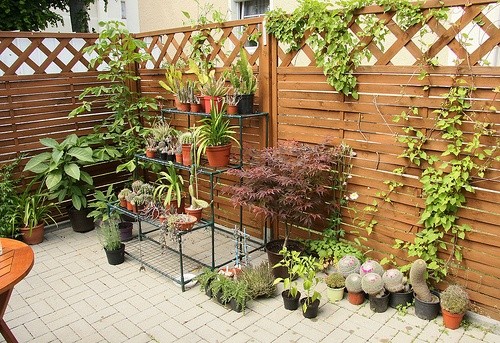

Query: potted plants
[8,20,471,330]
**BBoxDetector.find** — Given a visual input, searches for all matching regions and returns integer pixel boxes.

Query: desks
[0,237,34,343]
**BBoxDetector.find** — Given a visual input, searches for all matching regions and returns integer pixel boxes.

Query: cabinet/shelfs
[106,107,269,292]
